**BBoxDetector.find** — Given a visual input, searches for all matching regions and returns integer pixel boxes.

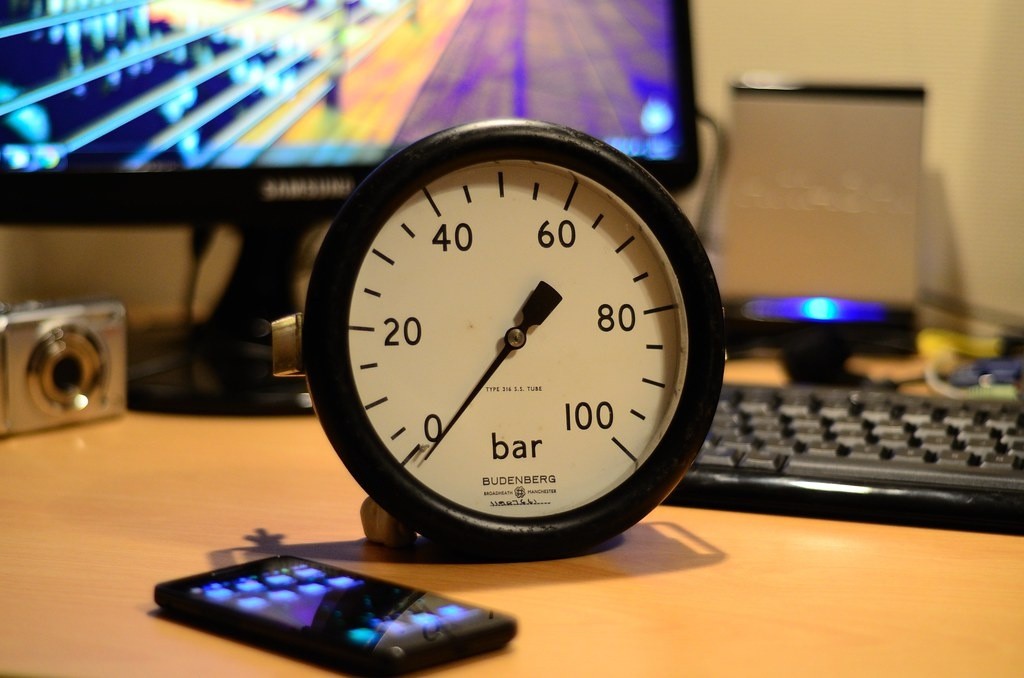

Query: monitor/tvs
[0,0,703,419]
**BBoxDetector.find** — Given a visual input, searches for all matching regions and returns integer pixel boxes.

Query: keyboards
[659,383,1024,538]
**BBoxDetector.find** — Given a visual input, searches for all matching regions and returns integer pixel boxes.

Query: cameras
[0,299,127,436]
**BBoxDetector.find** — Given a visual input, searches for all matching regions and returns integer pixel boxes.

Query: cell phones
[154,555,517,678]
